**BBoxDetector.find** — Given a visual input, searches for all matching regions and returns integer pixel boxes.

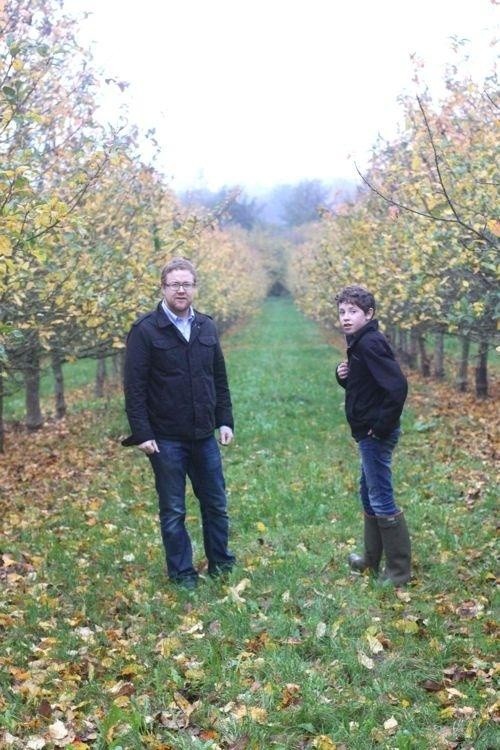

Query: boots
[377,508,412,589]
[347,514,383,574]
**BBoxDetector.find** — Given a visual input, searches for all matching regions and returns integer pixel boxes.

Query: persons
[335,284,414,594]
[119,257,238,590]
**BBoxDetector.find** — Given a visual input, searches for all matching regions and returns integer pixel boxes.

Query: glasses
[162,281,196,291]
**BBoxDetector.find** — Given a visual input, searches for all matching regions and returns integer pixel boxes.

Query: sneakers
[168,569,233,593]
